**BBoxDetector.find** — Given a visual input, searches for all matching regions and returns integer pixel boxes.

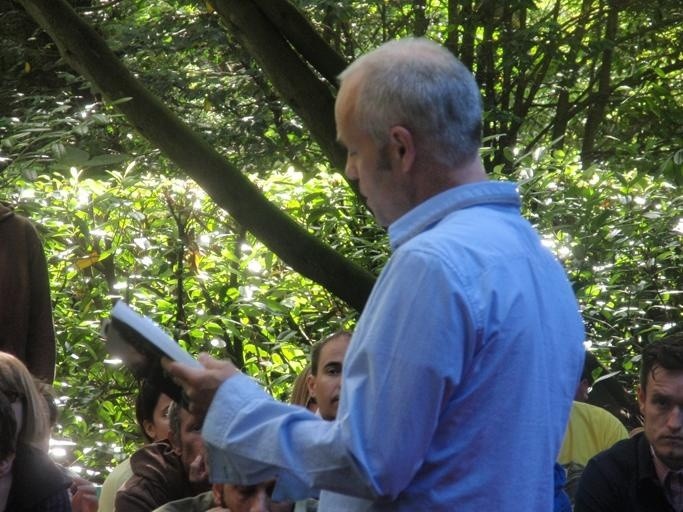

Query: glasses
[3,389,21,403]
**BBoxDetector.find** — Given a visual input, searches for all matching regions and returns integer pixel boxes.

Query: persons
[161,36,586,512]
[1,199,683,511]
